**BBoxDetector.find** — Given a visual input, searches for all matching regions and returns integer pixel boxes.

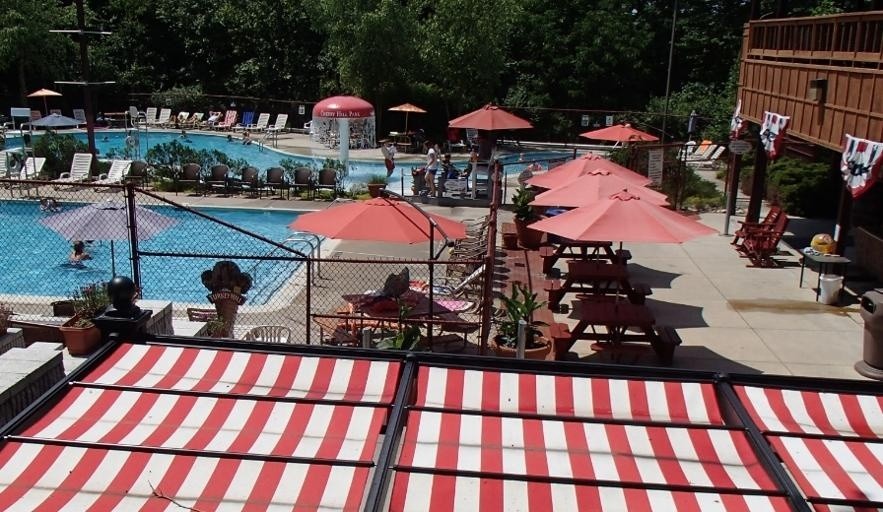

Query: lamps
[809,77,828,104]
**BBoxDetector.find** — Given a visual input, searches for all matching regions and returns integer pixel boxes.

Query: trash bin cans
[855,288,883,381]
[818,274,844,305]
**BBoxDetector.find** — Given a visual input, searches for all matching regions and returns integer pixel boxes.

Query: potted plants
[507,179,550,255]
[481,276,553,363]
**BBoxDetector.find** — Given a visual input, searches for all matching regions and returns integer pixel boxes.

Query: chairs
[174,163,201,196]
[196,112,221,131]
[260,168,284,198]
[151,108,172,130]
[122,160,148,195]
[673,136,726,175]
[5,157,47,189]
[231,112,254,133]
[264,113,288,134]
[89,158,132,193]
[240,325,294,344]
[30,112,42,131]
[167,111,189,129]
[328,104,511,358]
[230,166,258,197]
[246,112,270,134]
[51,152,92,192]
[181,113,205,129]
[727,203,782,252]
[287,168,312,200]
[50,109,61,115]
[313,168,336,200]
[736,209,792,269]
[213,110,238,131]
[205,165,228,197]
[129,106,139,126]
[74,109,87,129]
[137,107,157,128]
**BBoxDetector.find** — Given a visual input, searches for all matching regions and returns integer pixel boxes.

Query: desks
[793,245,852,304]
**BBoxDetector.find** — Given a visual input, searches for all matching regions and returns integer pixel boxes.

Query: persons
[227,135,234,142]
[39,198,53,212]
[387,142,397,158]
[379,140,395,177]
[469,146,480,163]
[48,198,63,213]
[423,140,438,197]
[442,154,460,179]
[167,111,219,129]
[241,131,251,145]
[70,242,91,261]
[178,131,187,137]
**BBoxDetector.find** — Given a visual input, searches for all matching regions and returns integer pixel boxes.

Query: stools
[538,230,684,365]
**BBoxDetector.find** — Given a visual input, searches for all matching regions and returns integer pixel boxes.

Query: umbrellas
[37,200,178,278]
[31,113,85,132]
[579,123,661,154]
[524,150,654,188]
[26,88,64,117]
[285,187,465,354]
[528,187,722,309]
[526,167,671,207]
[387,102,428,154]
[447,102,535,164]
[686,109,698,141]
